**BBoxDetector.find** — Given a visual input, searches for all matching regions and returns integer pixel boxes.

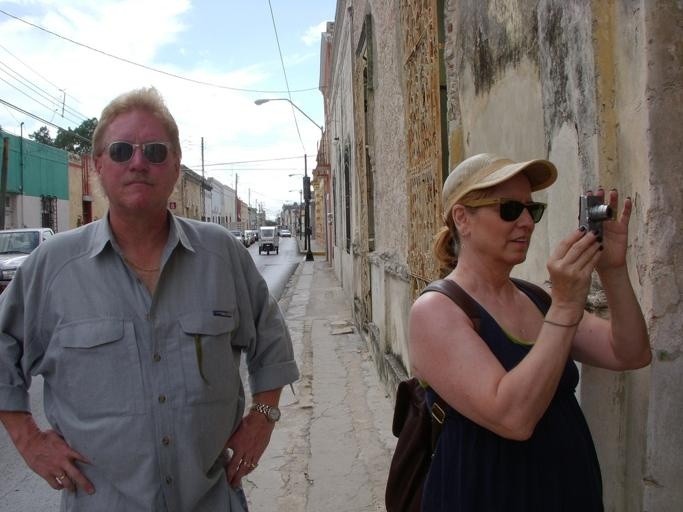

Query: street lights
[254,97,329,265]
[288,173,304,178]
[285,189,302,238]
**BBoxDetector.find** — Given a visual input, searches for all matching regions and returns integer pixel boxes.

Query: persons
[0,91,300,512]
[408,152,653,511]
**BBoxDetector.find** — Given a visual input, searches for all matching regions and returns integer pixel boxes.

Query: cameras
[579,195,612,250]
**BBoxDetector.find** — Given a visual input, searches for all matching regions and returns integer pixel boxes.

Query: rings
[242,461,250,467]
[251,464,255,468]
[59,474,65,480]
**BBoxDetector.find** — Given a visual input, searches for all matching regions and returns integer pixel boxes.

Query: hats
[440,152,557,224]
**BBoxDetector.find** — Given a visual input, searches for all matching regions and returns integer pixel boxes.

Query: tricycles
[258,226,278,256]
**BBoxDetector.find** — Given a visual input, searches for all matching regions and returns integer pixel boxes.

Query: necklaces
[124,257,160,272]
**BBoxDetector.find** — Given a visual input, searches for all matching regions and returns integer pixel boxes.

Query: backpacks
[385,277,552,512]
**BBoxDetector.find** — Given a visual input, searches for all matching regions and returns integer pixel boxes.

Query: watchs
[248,402,281,422]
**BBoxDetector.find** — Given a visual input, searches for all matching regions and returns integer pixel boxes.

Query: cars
[0,228,56,289]
[280,230,290,237]
[228,229,260,248]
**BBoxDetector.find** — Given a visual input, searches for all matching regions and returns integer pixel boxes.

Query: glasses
[463,196,548,223]
[97,141,171,165]
